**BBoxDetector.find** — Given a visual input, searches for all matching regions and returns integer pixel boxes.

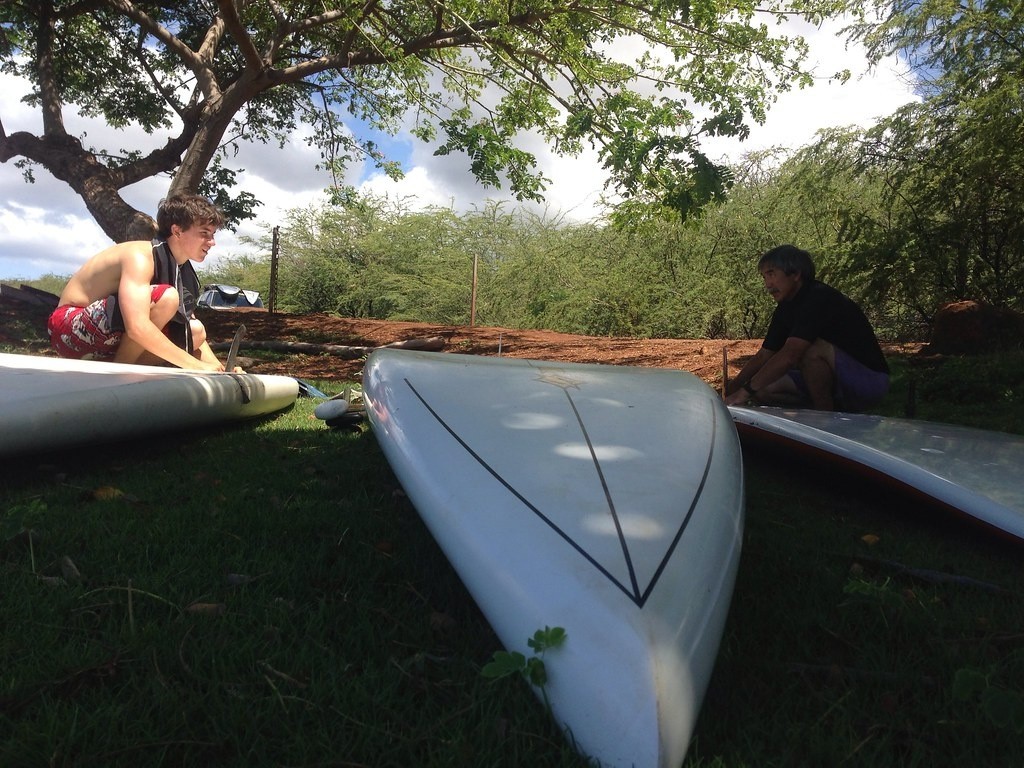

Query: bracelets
[742,380,758,395]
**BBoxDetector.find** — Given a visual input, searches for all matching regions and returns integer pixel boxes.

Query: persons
[726,246,890,411]
[48,194,246,374]
